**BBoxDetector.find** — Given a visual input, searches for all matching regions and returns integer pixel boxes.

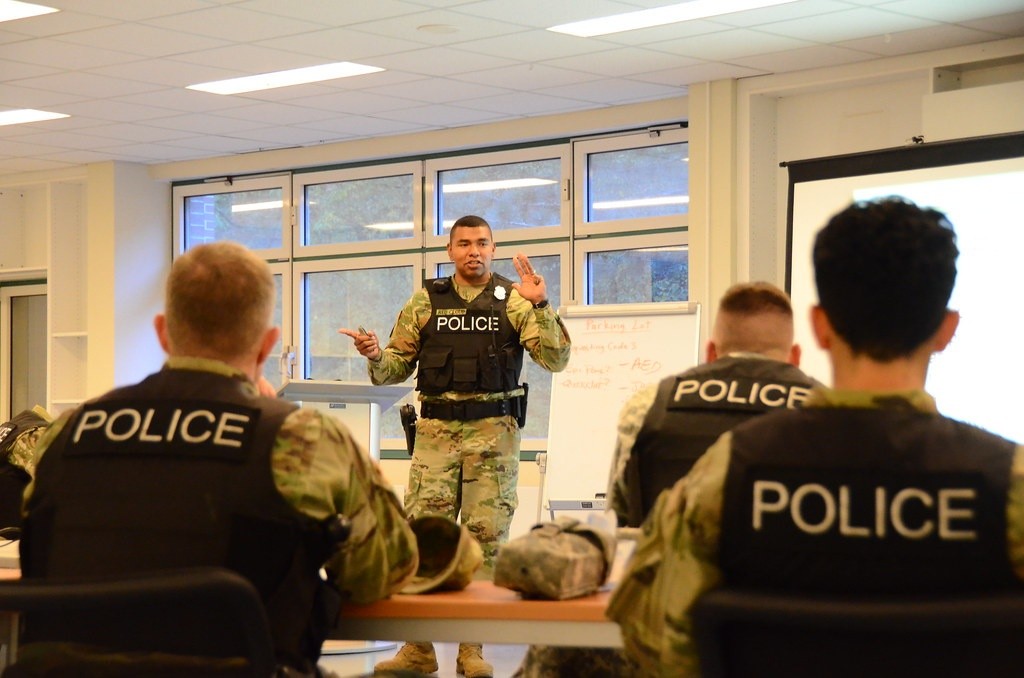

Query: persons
[340,215,571,678]
[0,405,54,541]
[605,281,826,529]
[605,196,1024,678]
[0,243,419,678]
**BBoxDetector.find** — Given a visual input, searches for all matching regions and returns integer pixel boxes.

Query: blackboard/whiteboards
[544,300,702,514]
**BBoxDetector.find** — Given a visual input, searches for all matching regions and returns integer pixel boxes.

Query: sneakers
[373,640,441,675]
[455,641,494,678]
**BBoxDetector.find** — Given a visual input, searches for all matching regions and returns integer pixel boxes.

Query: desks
[0,541,639,645]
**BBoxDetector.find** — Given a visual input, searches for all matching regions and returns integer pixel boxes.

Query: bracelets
[533,299,548,309]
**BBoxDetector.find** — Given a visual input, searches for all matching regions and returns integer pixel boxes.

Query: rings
[531,270,536,275]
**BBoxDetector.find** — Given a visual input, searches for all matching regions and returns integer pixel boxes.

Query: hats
[396,512,482,596]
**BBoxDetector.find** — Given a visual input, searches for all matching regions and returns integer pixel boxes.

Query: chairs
[0,572,278,677]
[688,591,1024,678]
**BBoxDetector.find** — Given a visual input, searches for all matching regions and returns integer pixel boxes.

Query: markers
[594,493,607,499]
